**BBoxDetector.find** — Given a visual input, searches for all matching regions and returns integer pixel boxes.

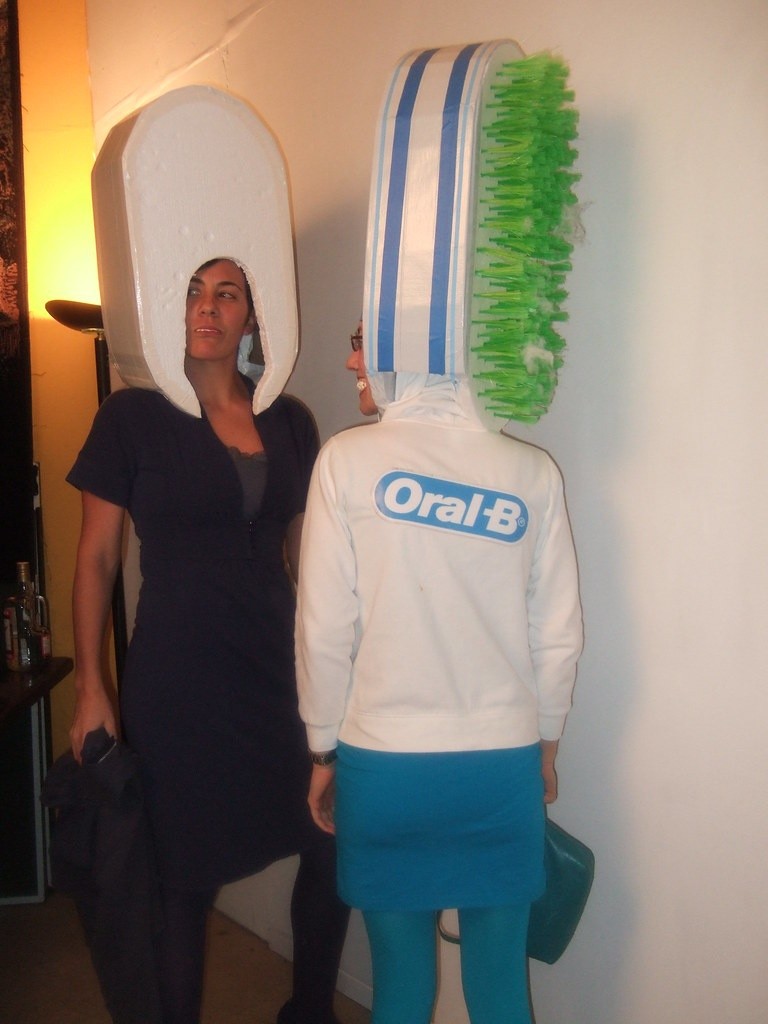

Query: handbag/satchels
[437,796,593,965]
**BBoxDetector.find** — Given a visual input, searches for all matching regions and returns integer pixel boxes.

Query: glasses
[350,330,362,352]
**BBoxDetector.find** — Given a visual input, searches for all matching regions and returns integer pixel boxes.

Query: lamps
[45,299,126,691]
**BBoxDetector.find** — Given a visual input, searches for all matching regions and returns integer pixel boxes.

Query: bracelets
[307,749,337,765]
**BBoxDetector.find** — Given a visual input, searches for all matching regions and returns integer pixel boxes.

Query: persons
[66,255,353,1024]
[293,318,581,1024]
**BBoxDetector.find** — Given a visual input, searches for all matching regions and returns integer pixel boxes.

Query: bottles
[3,562,51,672]
